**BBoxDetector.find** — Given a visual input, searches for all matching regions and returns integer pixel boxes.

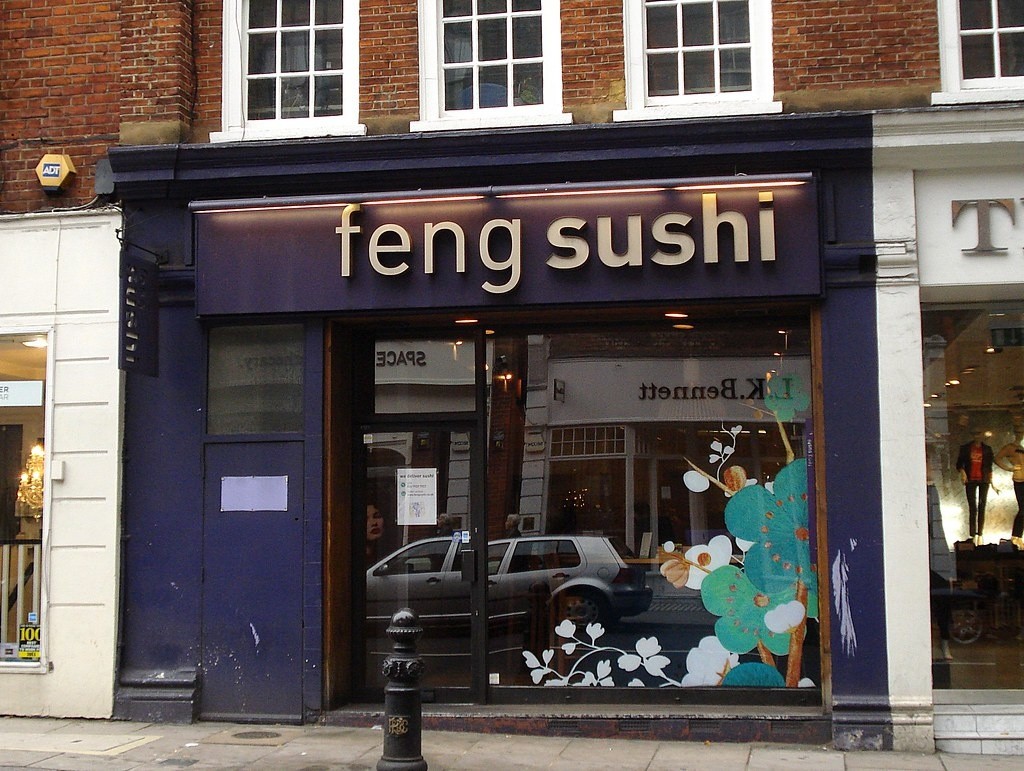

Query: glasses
[970,432,982,435]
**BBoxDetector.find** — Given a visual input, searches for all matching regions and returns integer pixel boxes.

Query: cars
[364,537,477,636]
[488,534,652,633]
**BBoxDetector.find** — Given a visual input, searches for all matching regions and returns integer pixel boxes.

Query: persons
[438,513,452,537]
[923,457,954,660]
[993,425,1024,550]
[956,427,1000,545]
[366,503,385,562]
[505,514,522,537]
